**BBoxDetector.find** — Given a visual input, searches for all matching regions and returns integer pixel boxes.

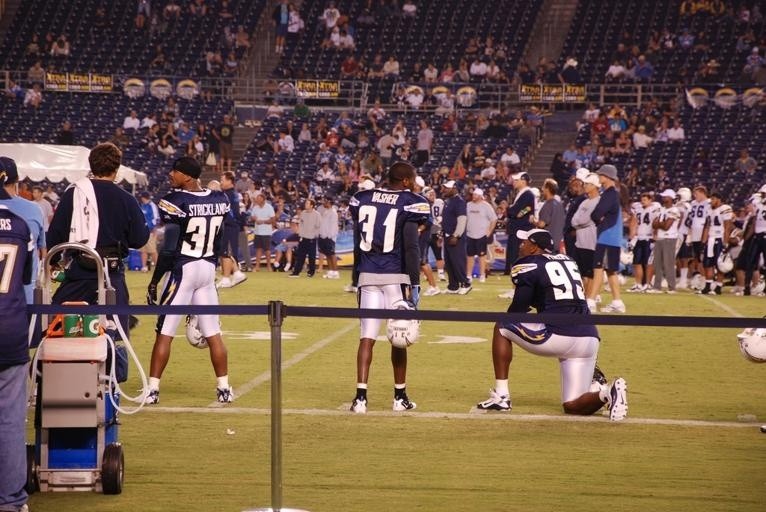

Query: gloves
[147,283,157,305]
[407,285,421,307]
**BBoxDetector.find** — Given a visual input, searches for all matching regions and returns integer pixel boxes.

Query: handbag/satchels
[78,243,123,272]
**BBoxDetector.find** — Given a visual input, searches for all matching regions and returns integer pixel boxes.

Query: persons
[347,163,432,414]
[45,143,150,384]
[0,2,764,314]
[477,229,628,421]
[0,157,46,510]
[134,156,234,405]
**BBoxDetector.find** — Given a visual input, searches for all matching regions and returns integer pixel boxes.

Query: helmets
[620,249,634,265]
[677,187,692,201]
[386,300,421,348]
[737,328,766,362]
[185,315,222,348]
[590,366,608,393]
[717,249,733,274]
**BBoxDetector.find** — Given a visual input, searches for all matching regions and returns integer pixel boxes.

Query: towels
[65,178,99,259]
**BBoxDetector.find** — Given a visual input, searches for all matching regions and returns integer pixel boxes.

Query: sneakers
[586,295,625,313]
[439,272,445,281]
[467,274,485,283]
[499,290,514,298]
[217,277,231,288]
[731,286,765,297]
[608,377,628,421]
[241,266,339,279]
[477,391,512,412]
[423,285,472,296]
[349,398,368,413]
[626,284,720,298]
[135,386,159,404]
[231,270,247,287]
[216,385,232,403]
[393,397,416,410]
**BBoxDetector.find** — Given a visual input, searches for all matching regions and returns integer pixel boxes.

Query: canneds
[83,314,100,337]
[64,314,82,337]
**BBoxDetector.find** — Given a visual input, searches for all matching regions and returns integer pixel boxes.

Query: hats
[173,156,201,178]
[576,164,618,188]
[415,175,485,200]
[659,189,676,199]
[516,228,554,253]
[357,179,376,190]
[0,161,13,200]
[511,171,529,180]
